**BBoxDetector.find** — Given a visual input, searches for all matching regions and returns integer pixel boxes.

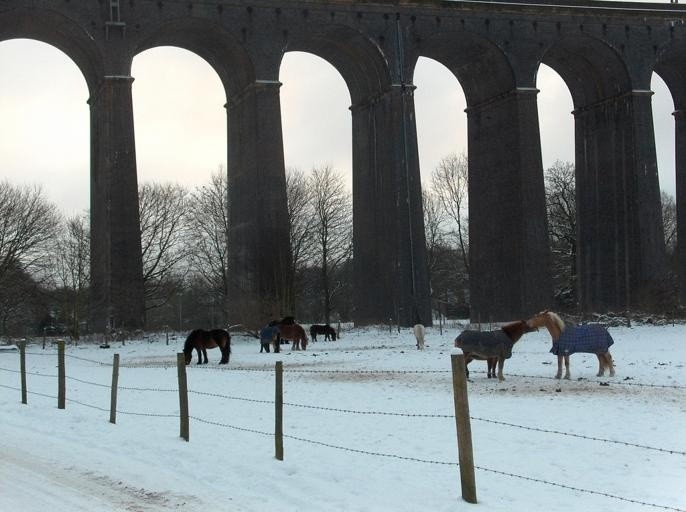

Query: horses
[528,308,615,380]
[454,319,537,382]
[260,316,309,353]
[182,328,231,365]
[414,324,425,351]
[310,325,336,342]
